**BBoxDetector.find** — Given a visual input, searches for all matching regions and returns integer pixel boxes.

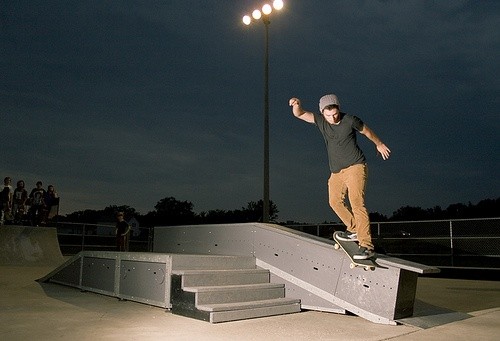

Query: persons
[27,181,47,217]
[288,94,391,260]
[12,178,28,214]
[115,211,132,251]
[0,177,14,220]
[42,185,58,224]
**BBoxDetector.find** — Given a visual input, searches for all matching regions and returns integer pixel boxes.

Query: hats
[118,212,123,216]
[318,95,341,115]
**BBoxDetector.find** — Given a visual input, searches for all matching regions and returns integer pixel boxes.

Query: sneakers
[353,246,376,260]
[336,231,358,242]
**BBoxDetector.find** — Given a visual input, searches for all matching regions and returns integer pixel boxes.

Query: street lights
[242,0,284,224]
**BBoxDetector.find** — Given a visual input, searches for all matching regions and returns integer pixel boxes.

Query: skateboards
[331,230,376,272]
